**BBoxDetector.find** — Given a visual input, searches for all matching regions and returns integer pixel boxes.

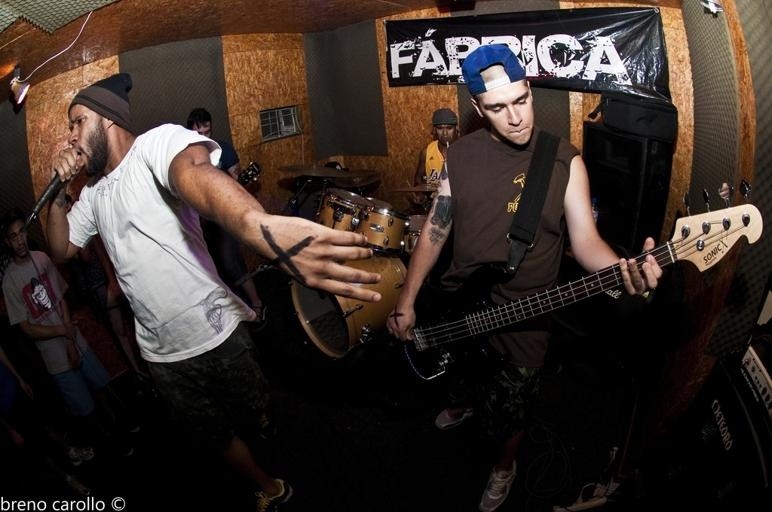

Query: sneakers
[64,445,97,468]
[255,477,294,512]
[477,458,517,512]
[435,406,473,431]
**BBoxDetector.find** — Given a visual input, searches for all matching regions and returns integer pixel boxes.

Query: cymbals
[336,169,382,187]
[278,165,353,178]
[393,185,436,192]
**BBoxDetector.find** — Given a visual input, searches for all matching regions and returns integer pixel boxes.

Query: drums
[290,251,407,357]
[318,188,427,254]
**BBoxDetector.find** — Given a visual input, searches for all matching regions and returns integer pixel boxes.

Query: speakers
[580,121,677,260]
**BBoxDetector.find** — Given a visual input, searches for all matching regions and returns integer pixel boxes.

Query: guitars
[391,204,763,383]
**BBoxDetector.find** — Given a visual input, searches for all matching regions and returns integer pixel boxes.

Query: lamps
[700,0,725,14]
[9,64,30,105]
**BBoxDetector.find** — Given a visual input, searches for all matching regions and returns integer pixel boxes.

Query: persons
[387,43,664,510]
[0,207,143,458]
[50,195,157,400]
[48,76,382,512]
[187,107,268,333]
[405,109,461,217]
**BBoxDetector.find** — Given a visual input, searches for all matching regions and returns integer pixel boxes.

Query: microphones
[31,148,82,219]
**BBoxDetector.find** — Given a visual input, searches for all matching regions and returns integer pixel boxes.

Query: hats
[67,72,136,135]
[431,107,457,127]
[461,44,526,95]
